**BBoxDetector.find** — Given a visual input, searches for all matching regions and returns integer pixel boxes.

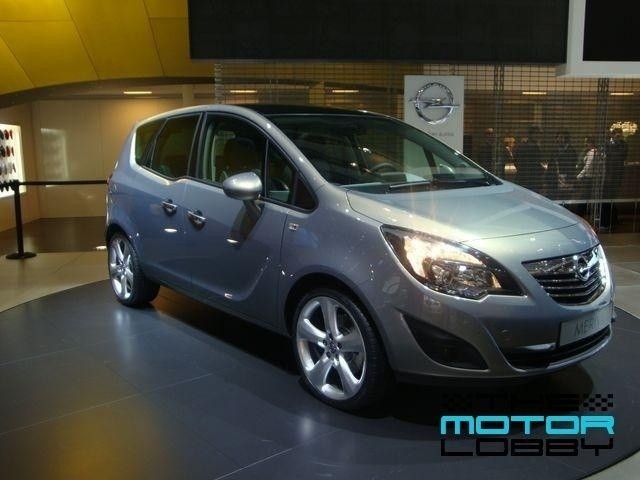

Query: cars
[104,104,618,414]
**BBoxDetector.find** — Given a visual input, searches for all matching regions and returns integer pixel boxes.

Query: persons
[482,122,628,232]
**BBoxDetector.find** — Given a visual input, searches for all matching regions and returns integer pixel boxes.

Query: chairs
[157,135,376,205]
